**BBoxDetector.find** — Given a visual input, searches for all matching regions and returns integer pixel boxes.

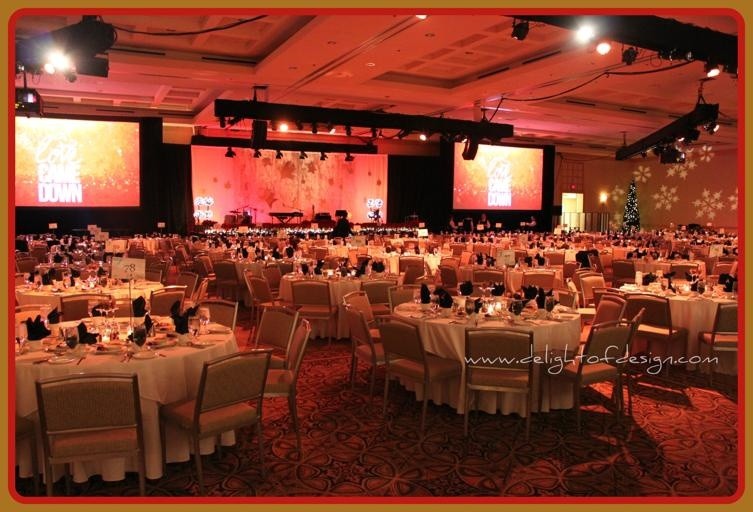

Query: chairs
[696,302,738,386]
[243,267,284,342]
[192,253,216,282]
[578,272,608,308]
[157,346,274,498]
[252,305,299,369]
[537,320,637,436]
[387,283,431,313]
[437,264,459,291]
[290,279,340,351]
[519,270,556,297]
[608,306,647,418]
[463,325,535,442]
[56,292,116,321]
[586,292,628,322]
[260,317,314,457]
[471,267,508,296]
[14,235,197,288]
[193,298,240,332]
[197,227,739,272]
[213,262,248,304]
[590,286,628,317]
[342,290,376,326]
[555,287,578,308]
[373,315,462,433]
[399,254,425,279]
[181,279,208,313]
[341,303,399,398]
[34,372,146,498]
[149,284,188,317]
[624,290,689,388]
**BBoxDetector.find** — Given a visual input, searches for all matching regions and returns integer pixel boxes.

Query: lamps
[225,147,236,157]
[345,153,354,162]
[272,119,436,142]
[704,62,721,77]
[511,18,530,41]
[320,153,327,161]
[299,152,308,159]
[621,48,639,67]
[276,151,284,159]
[253,149,261,157]
[461,138,480,161]
[640,123,717,163]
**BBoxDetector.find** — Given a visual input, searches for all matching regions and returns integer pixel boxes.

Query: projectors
[660,151,686,163]
[14,87,44,117]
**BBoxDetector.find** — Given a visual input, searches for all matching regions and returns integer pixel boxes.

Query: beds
[393,295,583,356]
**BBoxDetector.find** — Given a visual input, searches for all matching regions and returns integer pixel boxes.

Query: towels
[433,288,454,309]
[132,296,148,317]
[509,300,529,315]
[536,287,559,309]
[77,320,99,344]
[129,314,153,343]
[521,285,537,299]
[47,305,64,323]
[420,283,430,303]
[169,300,199,333]
[26,314,51,340]
[492,280,505,296]
[459,280,474,296]
[473,298,482,312]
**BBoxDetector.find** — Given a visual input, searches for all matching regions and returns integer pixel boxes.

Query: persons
[329,212,351,237]
[478,214,491,233]
[449,214,459,235]
[526,216,538,231]
[462,214,474,234]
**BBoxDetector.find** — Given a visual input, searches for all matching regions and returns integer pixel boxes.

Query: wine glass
[16,323,28,351]
[63,279,71,290]
[102,308,112,336]
[486,286,494,308]
[111,306,119,333]
[545,296,554,319]
[96,307,105,336]
[187,316,200,343]
[512,300,523,322]
[430,294,439,318]
[413,289,421,308]
[198,306,210,336]
[133,326,147,352]
[697,281,705,298]
[40,305,52,328]
[465,299,475,319]
[689,267,697,277]
[479,287,486,313]
[100,275,107,292]
[64,326,79,362]
